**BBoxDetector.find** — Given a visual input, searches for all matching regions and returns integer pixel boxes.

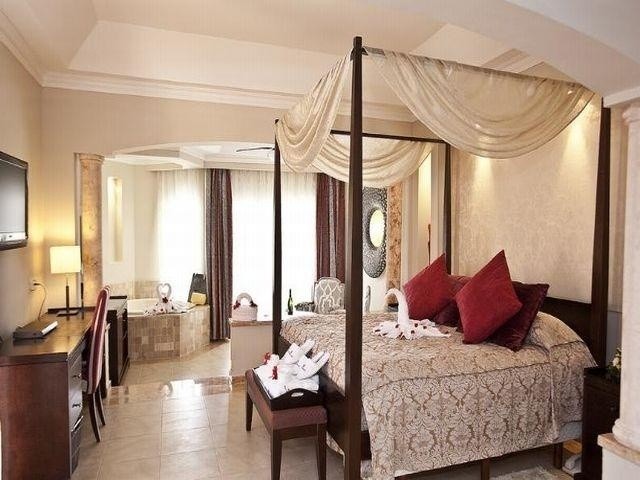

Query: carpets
[485,464,573,480]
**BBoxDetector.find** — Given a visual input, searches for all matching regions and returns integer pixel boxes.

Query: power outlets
[30,279,41,292]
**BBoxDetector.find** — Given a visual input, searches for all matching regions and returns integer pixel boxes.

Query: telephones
[562,454,582,475]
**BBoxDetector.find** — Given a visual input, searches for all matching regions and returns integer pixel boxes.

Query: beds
[273,296,611,480]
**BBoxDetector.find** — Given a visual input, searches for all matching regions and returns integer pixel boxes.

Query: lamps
[49,246,82,316]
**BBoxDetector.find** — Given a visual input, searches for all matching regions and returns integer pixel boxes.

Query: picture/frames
[361,185,388,277]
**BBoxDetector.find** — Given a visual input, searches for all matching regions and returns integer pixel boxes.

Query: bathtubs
[127,298,210,359]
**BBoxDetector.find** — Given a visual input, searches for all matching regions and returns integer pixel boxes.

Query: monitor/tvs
[0,150,29,251]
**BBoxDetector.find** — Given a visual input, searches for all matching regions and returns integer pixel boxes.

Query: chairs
[314,276,346,314]
[82,285,110,442]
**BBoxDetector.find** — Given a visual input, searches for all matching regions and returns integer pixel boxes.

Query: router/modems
[13,319,58,338]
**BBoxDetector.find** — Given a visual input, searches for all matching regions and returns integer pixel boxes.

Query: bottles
[288,291,294,316]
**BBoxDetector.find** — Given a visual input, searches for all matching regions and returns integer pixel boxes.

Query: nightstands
[581,367,620,479]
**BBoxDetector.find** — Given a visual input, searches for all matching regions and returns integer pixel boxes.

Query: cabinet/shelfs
[90,296,130,386]
[0,307,94,480]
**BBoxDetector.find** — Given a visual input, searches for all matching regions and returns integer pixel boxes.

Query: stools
[245,369,328,480]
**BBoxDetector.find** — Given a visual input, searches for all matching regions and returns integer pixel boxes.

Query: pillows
[403,250,550,351]
